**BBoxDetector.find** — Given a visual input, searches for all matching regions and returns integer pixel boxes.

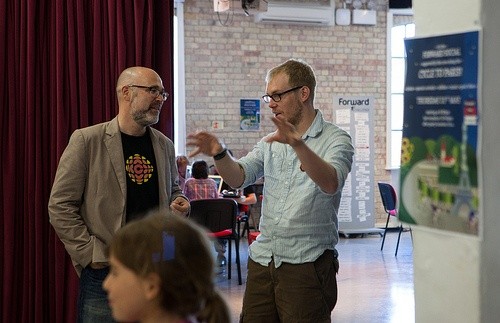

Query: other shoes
[216,259,226,267]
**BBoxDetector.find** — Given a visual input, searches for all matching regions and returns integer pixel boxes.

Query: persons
[209,163,265,198]
[176,156,191,194]
[47,66,191,322]
[217,184,258,218]
[184,160,227,267]
[186,59,354,322]
[101,210,231,323]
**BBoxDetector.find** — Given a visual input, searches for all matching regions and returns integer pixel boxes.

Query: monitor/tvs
[208,175,223,193]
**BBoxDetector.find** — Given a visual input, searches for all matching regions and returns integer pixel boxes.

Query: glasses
[262,86,302,104]
[127,84,169,101]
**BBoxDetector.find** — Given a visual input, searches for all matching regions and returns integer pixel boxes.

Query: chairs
[377,181,413,256]
[236,184,263,269]
[189,198,243,286]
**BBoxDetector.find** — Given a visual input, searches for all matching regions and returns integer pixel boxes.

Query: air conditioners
[254,0,333,26]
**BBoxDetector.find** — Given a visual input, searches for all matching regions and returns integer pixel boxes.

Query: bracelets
[213,148,228,160]
